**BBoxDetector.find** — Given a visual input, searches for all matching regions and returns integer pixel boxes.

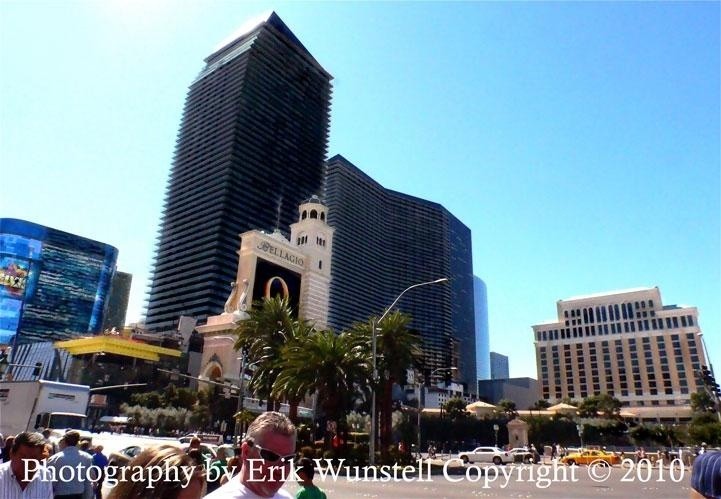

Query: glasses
[252,444,297,465]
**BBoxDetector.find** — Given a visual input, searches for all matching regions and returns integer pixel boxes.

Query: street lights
[211,333,244,450]
[697,332,718,400]
[415,363,459,464]
[369,272,447,473]
[493,424,499,447]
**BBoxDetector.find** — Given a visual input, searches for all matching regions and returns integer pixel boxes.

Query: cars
[115,444,146,464]
[459,445,620,470]
[175,432,238,469]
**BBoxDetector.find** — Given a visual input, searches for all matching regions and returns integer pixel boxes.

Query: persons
[689,452,721,499]
[198,411,295,499]
[0,422,244,499]
[294,456,326,498]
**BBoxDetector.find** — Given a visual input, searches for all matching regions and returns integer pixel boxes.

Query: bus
[347,431,370,450]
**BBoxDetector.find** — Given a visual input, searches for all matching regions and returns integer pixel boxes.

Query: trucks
[0,379,96,448]
